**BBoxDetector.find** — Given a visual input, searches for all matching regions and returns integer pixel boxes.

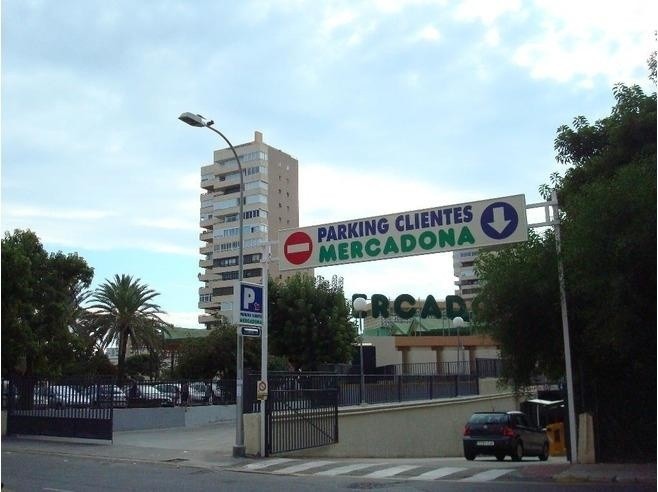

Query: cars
[2,374,224,411]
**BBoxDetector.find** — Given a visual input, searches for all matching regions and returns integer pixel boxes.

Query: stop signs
[283,231,314,267]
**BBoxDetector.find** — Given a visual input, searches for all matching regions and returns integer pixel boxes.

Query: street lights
[177,111,246,459]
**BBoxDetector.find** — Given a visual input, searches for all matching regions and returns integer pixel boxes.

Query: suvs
[463,409,550,461]
